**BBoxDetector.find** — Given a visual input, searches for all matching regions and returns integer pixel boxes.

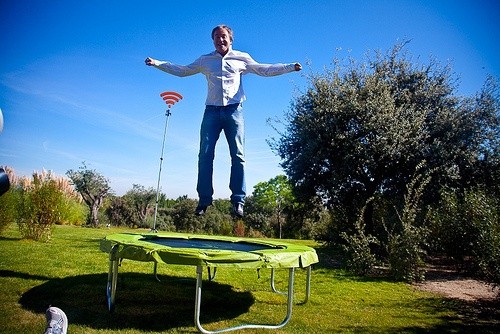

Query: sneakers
[43,307,68,334]
[195,200,212,216]
[231,202,243,217]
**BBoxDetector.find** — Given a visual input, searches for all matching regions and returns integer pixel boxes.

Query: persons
[145,24,303,218]
